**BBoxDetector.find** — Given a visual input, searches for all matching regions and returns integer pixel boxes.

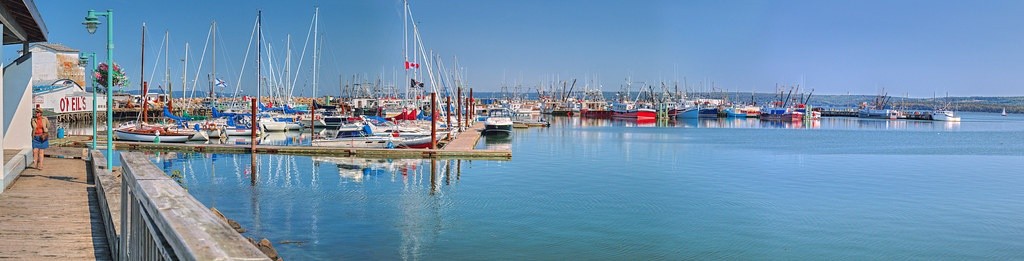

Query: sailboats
[487,69,963,123]
[1000,106,1008,117]
[114,1,480,147]
[311,156,424,198]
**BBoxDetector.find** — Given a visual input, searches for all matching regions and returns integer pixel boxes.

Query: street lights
[81,7,115,171]
[79,50,98,151]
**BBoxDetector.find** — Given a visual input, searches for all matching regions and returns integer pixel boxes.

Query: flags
[410,79,424,89]
[216,78,228,88]
[405,61,419,70]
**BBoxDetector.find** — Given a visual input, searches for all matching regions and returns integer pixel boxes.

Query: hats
[36,108,43,113]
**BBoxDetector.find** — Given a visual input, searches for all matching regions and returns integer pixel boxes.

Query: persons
[31,107,50,170]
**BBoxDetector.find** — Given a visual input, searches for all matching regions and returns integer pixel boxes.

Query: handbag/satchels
[41,133,47,141]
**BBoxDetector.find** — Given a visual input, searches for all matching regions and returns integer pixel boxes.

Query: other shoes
[32,165,43,170]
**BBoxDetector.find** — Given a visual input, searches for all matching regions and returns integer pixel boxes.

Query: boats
[484,108,514,135]
